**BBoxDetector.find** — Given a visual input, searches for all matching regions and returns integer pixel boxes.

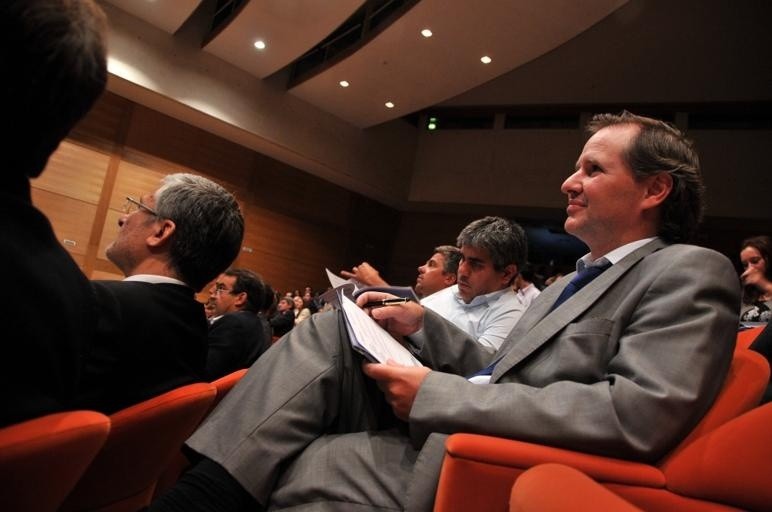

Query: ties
[456,265,610,381]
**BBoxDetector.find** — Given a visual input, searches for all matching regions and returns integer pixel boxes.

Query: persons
[87,170,255,425]
[1,1,111,436]
[146,108,741,512]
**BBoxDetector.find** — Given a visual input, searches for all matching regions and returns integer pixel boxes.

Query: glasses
[121,195,158,218]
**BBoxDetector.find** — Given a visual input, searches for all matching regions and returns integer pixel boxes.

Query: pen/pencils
[364,297,408,307]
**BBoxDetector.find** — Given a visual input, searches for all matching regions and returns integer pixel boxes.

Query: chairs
[1,333,280,512]
[430,326,772,511]
